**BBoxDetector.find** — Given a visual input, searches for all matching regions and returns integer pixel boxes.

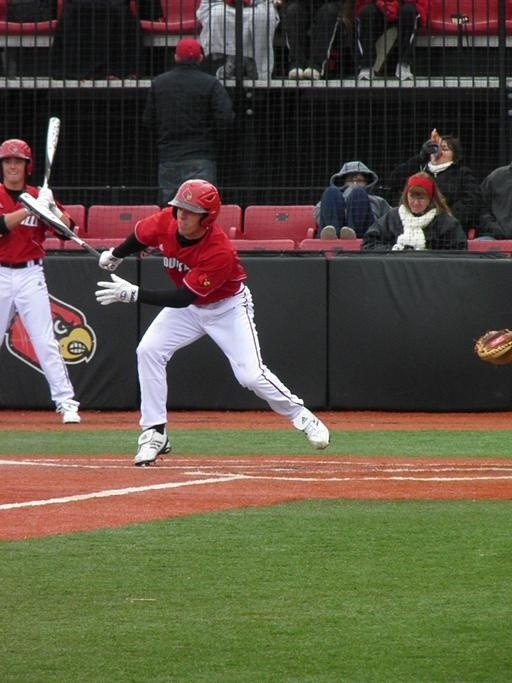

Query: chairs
[0,0,63,49]
[417,1,512,49]
[0,201,512,261]
[132,0,200,48]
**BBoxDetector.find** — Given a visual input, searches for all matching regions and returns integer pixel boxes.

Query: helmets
[0,138,33,175]
[167,178,220,231]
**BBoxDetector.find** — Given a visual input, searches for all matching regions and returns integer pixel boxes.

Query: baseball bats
[44,117,60,187]
[16,191,116,271]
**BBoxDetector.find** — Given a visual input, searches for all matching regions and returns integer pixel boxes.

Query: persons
[94,178,331,466]
[48,0,167,81]
[475,161,511,241]
[0,137,86,423]
[278,1,347,78]
[138,37,237,208]
[311,159,392,244]
[360,171,468,250]
[388,133,479,236]
[192,0,282,84]
[353,0,430,82]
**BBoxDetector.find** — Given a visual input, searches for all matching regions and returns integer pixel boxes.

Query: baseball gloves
[474,328,512,365]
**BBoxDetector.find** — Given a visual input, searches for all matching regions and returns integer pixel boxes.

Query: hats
[175,39,201,59]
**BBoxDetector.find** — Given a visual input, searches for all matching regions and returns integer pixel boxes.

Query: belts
[1,259,38,268]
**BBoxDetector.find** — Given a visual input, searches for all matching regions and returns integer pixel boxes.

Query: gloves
[36,196,50,209]
[98,247,124,271]
[95,273,139,305]
[420,139,438,162]
[39,186,56,205]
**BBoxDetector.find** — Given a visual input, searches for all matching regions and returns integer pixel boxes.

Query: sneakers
[320,225,338,239]
[62,410,81,423]
[291,406,330,449]
[340,226,357,239]
[358,66,375,79]
[289,66,319,79]
[395,63,414,80]
[134,426,171,464]
